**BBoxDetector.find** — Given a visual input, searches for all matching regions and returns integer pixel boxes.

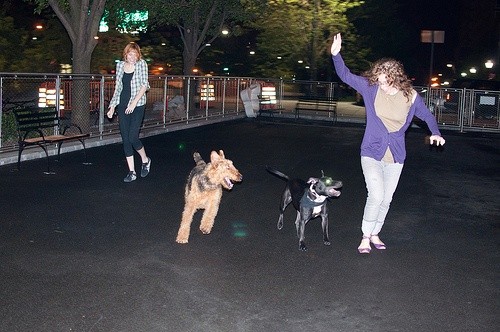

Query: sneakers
[141,157,151,177]
[124,171,137,182]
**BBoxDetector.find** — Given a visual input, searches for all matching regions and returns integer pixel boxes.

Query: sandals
[370,235,387,250]
[358,237,371,254]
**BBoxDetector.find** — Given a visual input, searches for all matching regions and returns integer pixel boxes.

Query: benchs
[257,108,285,122]
[14,107,93,176]
[294,96,338,126]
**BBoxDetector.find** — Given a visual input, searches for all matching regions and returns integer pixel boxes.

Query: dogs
[176,150,243,244]
[264,163,343,251]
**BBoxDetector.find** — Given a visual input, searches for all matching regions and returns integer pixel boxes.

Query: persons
[106,42,152,182]
[330,32,445,254]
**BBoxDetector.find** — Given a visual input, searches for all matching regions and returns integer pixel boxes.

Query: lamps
[221,26,230,36]
